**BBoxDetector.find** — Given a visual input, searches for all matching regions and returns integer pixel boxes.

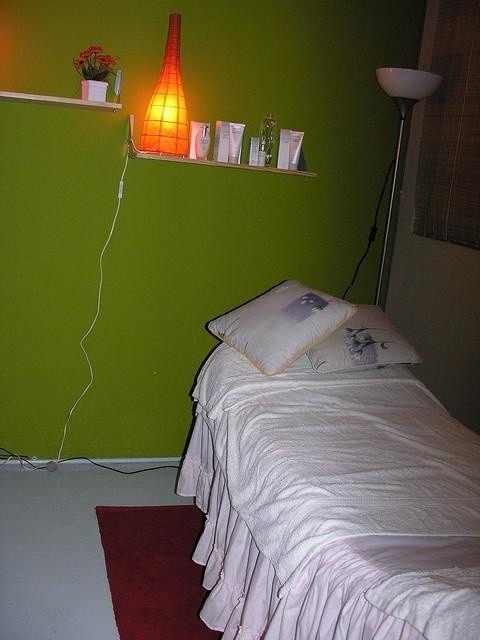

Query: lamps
[373,66,446,305]
[138,12,191,157]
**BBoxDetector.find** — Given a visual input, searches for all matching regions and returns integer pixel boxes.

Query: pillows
[205,278,425,376]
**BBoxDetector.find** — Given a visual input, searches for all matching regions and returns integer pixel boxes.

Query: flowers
[73,45,118,81]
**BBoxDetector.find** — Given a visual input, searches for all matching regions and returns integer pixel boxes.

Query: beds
[181,343,479,640]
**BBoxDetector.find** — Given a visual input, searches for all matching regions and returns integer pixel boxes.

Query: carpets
[96,503,229,640]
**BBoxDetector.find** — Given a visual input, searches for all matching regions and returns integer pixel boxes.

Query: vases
[80,79,110,102]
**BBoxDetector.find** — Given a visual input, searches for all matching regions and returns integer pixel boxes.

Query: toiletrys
[248,137,266,167]
[277,129,304,169]
[189,120,246,171]
